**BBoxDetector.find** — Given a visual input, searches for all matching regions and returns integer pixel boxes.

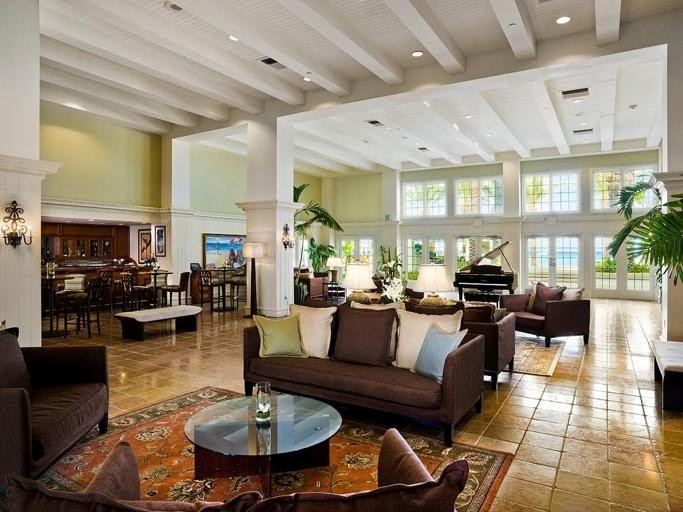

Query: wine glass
[144,253,155,268]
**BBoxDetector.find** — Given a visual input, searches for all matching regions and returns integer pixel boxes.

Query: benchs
[651,340,683,412]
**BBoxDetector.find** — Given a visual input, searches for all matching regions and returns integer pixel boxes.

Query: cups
[256,422,271,455]
[254,382,272,423]
[47,262,55,279]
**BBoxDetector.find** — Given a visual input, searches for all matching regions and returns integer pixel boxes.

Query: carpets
[33,385,515,511]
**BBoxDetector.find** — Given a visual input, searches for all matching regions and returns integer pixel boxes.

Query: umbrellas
[229,237,243,244]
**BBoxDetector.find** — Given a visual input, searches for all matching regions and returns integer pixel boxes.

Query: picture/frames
[202,233,247,277]
[137,225,166,264]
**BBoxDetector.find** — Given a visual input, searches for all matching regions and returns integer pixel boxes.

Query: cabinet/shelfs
[61,237,113,261]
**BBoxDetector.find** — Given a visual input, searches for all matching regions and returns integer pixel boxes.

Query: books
[63,277,84,290]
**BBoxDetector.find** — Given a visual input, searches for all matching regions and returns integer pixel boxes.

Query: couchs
[0,326,110,482]
[4,428,468,512]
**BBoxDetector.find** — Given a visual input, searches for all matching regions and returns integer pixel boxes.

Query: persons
[215,241,221,255]
[228,247,236,260]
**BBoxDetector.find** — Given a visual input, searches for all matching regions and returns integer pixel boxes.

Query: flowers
[370,244,415,307]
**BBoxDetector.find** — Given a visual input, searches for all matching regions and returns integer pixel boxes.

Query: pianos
[454,241,518,300]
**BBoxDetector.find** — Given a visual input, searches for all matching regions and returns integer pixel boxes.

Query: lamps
[0,199,33,249]
[329,258,343,284]
[280,224,295,249]
[242,243,264,318]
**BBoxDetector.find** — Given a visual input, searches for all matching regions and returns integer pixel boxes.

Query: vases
[371,275,406,291]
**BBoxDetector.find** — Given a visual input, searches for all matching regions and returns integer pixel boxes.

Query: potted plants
[293,183,344,304]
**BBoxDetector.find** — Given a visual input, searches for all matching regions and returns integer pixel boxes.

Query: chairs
[41,262,246,339]
